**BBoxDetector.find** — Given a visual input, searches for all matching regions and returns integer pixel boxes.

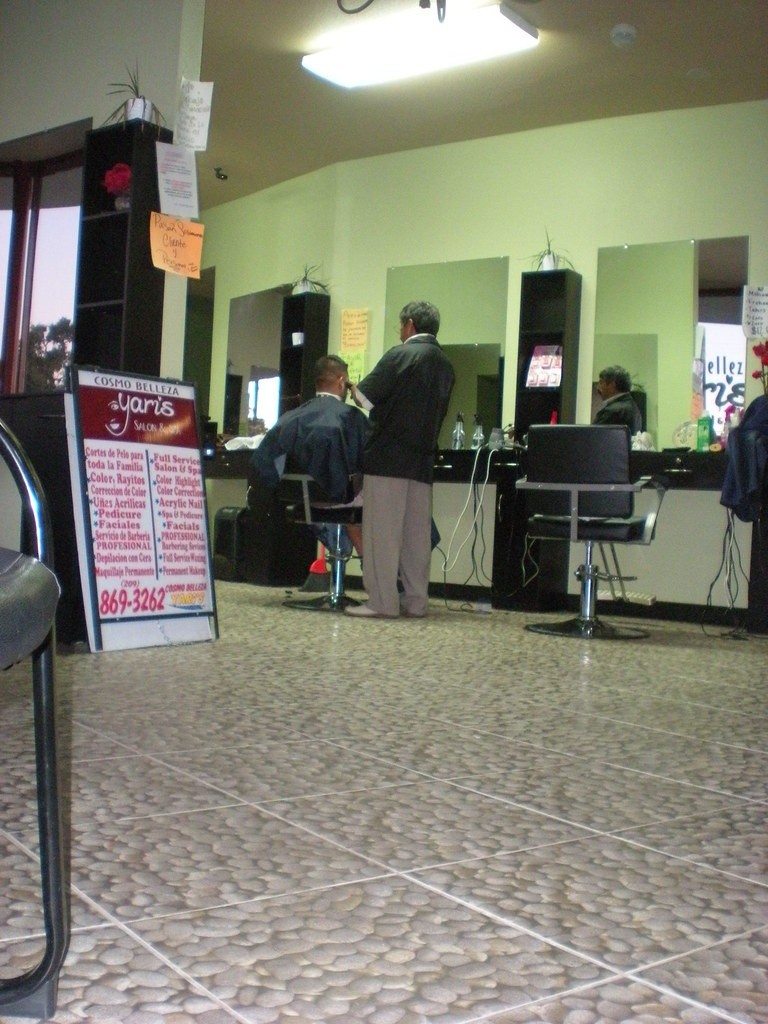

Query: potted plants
[290,267,322,293]
[529,232,572,271]
[95,57,160,120]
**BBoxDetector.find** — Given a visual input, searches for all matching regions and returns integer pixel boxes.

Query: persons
[591,365,642,436]
[251,355,369,571]
[345,301,456,616]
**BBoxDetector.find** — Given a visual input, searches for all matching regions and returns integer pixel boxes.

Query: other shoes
[346,604,398,619]
[400,605,423,619]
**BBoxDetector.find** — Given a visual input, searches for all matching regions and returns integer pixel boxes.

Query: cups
[291,332,305,346]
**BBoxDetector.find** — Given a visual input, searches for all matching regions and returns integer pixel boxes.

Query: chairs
[277,473,364,612]
[514,423,670,642]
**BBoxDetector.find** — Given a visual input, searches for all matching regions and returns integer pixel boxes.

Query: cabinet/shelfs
[493,268,582,608]
[1,393,95,640]
[279,291,330,419]
[70,117,174,375]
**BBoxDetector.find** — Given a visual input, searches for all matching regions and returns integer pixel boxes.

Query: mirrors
[383,257,509,450]
[589,233,750,453]
[222,285,292,435]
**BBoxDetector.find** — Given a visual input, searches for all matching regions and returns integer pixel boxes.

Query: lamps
[301,0,538,88]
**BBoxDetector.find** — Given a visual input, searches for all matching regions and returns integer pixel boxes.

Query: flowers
[753,341,768,396]
